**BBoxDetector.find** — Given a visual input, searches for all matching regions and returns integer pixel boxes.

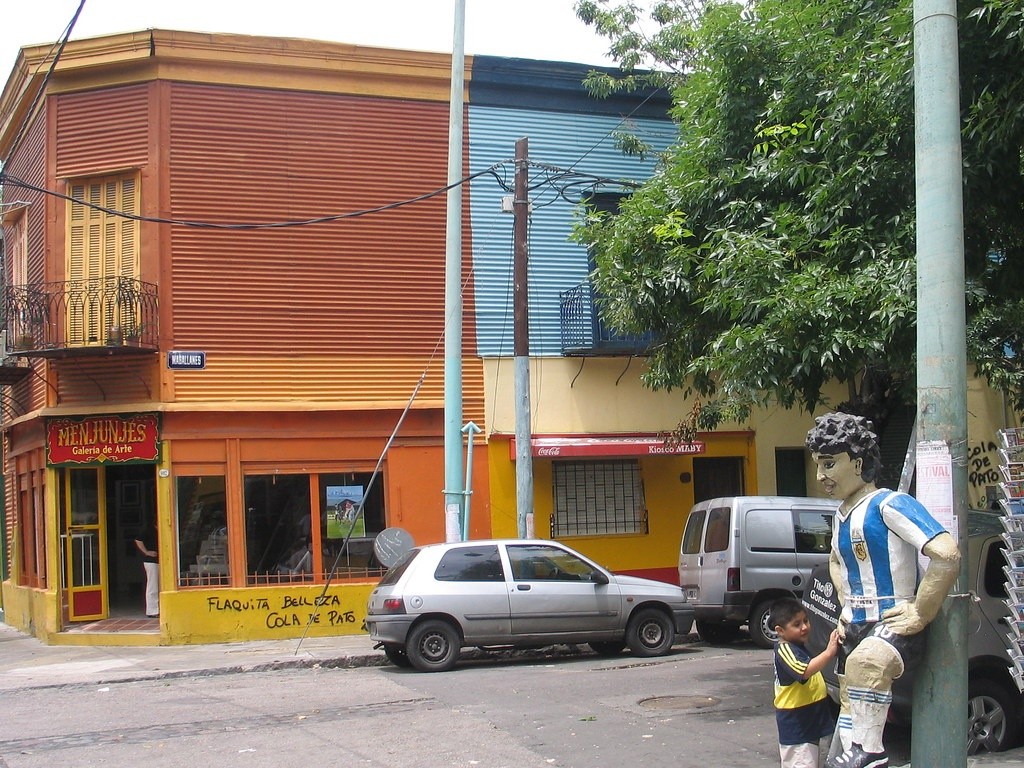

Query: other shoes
[148,615,159,618]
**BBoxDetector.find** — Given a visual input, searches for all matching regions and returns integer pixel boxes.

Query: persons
[768,597,840,768]
[133,519,160,619]
[804,410,963,768]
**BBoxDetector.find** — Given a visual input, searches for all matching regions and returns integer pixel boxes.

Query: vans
[676,495,842,647]
[807,510,1024,758]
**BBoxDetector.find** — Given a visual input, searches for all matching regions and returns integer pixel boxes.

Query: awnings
[508,436,709,458]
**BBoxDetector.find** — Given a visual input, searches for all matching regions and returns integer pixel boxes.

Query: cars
[363,538,696,673]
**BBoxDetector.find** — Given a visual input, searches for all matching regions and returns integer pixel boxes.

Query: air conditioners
[0,330,19,368]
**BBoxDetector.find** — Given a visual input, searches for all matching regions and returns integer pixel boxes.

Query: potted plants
[16,331,34,353]
[108,323,156,346]
